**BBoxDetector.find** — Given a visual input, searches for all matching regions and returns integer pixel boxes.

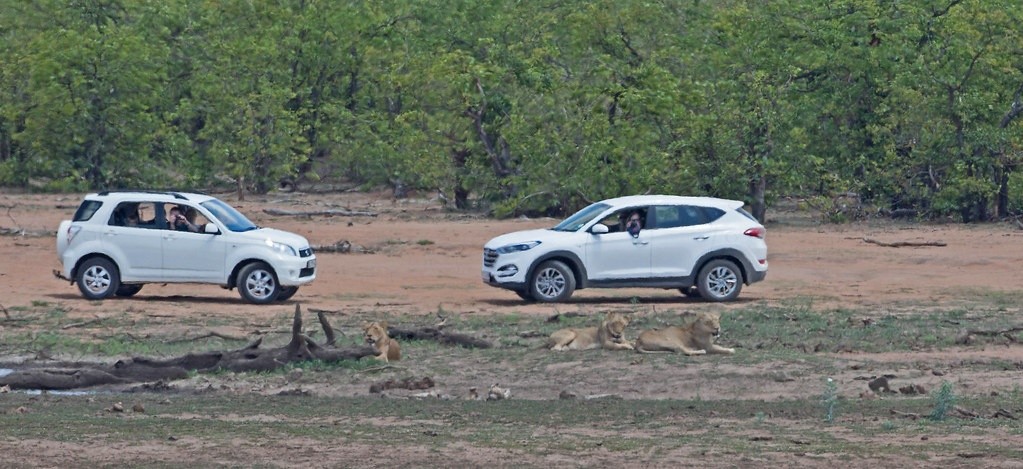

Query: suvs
[482,193,769,304]
[56,190,318,305]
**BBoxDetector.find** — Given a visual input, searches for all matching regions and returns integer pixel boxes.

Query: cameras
[630,219,637,229]
[176,215,185,220]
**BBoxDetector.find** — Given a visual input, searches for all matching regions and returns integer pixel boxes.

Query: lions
[361,321,400,363]
[635,311,735,356]
[544,311,636,352]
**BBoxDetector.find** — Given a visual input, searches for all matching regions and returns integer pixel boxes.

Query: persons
[625,211,642,231]
[167,207,201,234]
